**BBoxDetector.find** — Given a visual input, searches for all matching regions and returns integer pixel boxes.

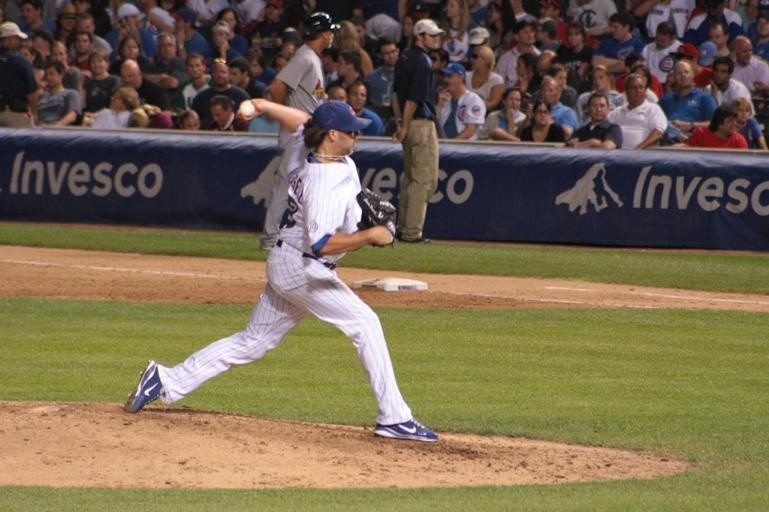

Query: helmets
[307,12,341,35]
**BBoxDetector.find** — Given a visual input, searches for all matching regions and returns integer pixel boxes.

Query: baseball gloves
[357,189,397,247]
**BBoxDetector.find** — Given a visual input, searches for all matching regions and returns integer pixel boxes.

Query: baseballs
[240,101,255,118]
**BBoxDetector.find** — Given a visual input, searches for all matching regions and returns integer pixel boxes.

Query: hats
[669,44,699,60]
[0,21,29,41]
[211,21,230,33]
[118,2,147,21]
[313,102,372,134]
[469,27,490,47]
[441,62,466,80]
[413,18,444,37]
[697,40,719,68]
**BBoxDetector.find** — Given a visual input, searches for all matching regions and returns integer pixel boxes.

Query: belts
[276,240,336,271]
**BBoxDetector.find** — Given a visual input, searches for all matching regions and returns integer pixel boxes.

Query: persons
[390,19,447,242]
[260,12,341,250]
[125,99,437,443]
[1,0,769,151]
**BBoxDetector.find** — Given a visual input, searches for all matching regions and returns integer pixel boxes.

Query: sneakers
[375,418,441,443]
[121,361,163,413]
